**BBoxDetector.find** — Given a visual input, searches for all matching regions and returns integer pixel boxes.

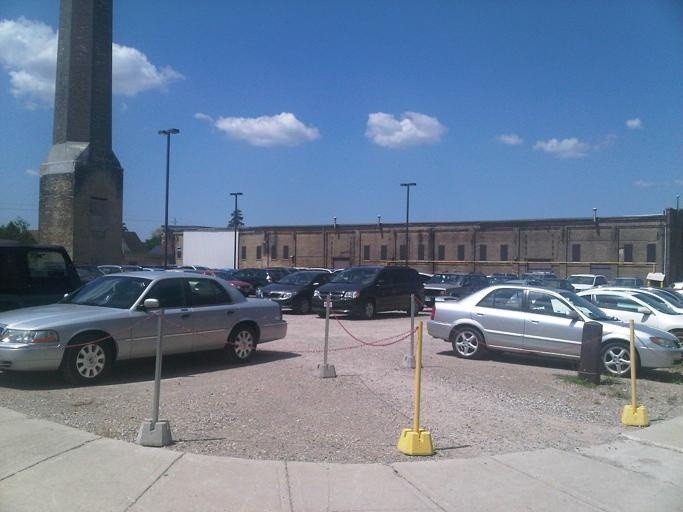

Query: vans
[0,244,83,311]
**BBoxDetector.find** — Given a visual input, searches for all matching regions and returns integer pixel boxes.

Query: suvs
[311,266,426,320]
[423,272,490,309]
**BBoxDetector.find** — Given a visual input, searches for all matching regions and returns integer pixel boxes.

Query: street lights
[400,182,417,267]
[158,128,180,266]
[230,193,243,269]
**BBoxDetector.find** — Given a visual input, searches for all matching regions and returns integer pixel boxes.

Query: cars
[74,263,344,298]
[0,271,288,389]
[486,268,683,346]
[255,270,337,315]
[427,284,683,377]
[418,272,445,286]
